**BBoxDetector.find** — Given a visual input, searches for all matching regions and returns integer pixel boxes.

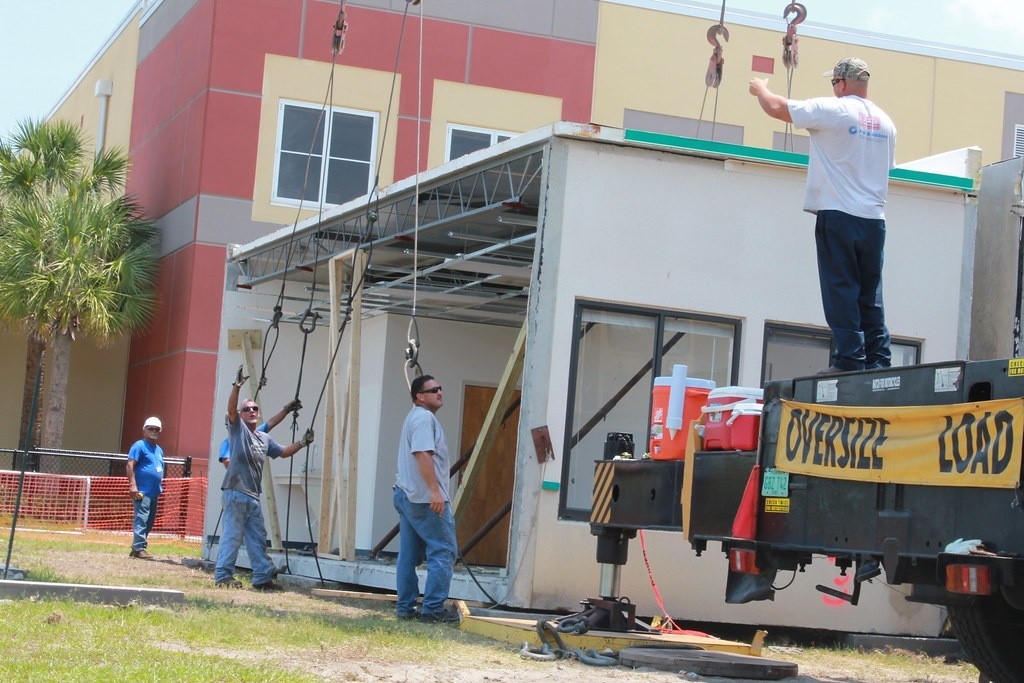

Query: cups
[499,567,507,577]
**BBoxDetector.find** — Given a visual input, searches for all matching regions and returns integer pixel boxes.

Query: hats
[822,57,872,80]
[143,416,161,430]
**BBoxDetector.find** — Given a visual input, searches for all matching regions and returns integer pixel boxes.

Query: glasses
[242,406,268,414]
[832,79,845,86]
[146,427,161,432]
[418,385,444,394]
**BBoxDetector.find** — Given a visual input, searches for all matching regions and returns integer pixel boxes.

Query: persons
[393,374,459,623]
[124,417,166,559]
[214,364,315,593]
[749,58,897,374]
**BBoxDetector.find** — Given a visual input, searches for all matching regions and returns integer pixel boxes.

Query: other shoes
[818,363,844,375]
[400,606,424,620]
[272,564,287,579]
[252,580,291,588]
[215,576,246,587]
[129,548,155,559]
[420,610,465,621]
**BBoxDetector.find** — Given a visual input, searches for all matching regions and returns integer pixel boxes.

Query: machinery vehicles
[552,354,1024,682]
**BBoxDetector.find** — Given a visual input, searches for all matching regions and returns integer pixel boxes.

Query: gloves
[284,398,304,413]
[233,363,254,389]
[301,428,316,446]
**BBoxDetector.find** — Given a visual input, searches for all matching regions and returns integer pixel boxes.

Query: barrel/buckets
[648,374,715,460]
[703,385,764,451]
